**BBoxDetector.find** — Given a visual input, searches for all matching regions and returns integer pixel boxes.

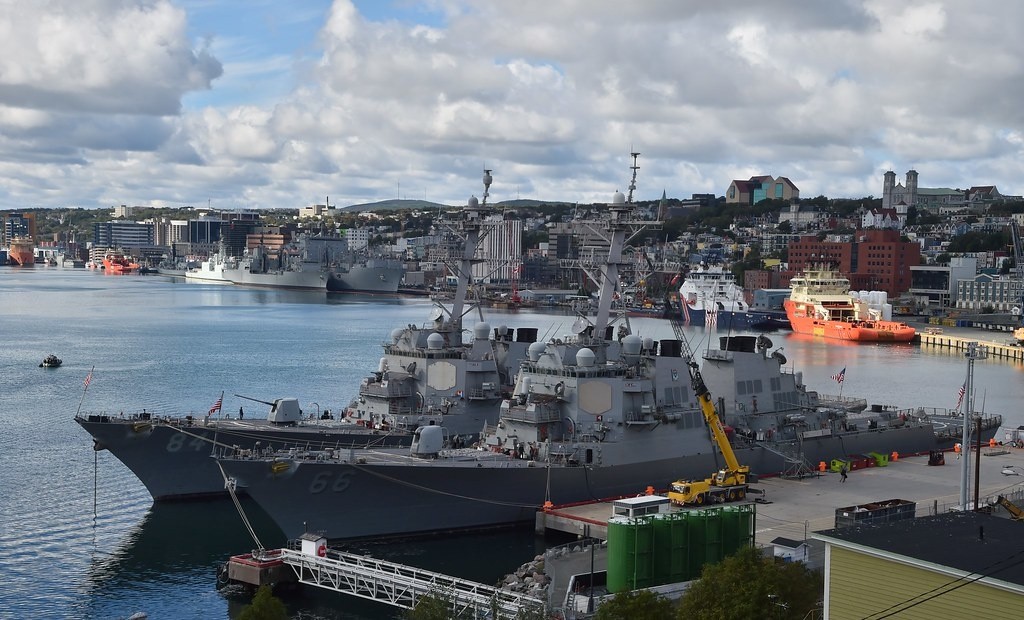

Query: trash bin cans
[831,459,849,474]
[849,452,889,471]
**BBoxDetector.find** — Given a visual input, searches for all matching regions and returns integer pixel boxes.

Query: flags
[955,383,965,410]
[84,369,93,386]
[208,396,222,416]
[831,368,844,383]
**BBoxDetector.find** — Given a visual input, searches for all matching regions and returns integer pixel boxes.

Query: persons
[839,466,846,483]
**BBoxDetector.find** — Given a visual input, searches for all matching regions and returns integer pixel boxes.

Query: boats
[267,224,407,292]
[783,263,915,342]
[73,167,868,503]
[209,146,1003,546]
[42,360,62,367]
[221,228,334,289]
[678,252,772,328]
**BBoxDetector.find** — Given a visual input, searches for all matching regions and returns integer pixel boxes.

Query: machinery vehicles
[643,251,772,506]
